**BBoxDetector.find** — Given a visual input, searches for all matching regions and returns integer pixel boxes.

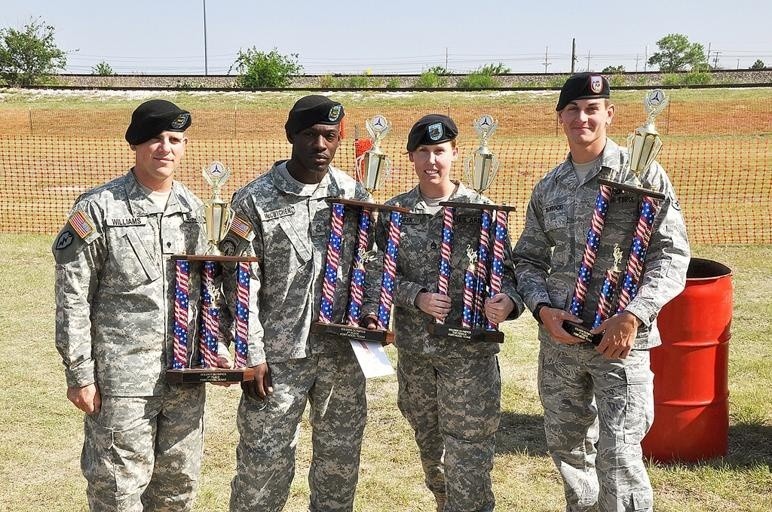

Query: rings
[492,313,496,319]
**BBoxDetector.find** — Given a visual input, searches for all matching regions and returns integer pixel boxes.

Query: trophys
[463,114,502,204]
[622,89,670,188]
[353,115,394,203]
[194,161,236,257]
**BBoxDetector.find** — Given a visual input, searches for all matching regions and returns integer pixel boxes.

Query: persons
[511,72,690,511]
[374,114,527,511]
[216,94,395,510]
[50,100,239,511]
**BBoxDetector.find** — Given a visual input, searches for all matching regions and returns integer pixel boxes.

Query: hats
[555,71,610,112]
[285,95,345,134]
[406,114,458,153]
[125,99,192,146]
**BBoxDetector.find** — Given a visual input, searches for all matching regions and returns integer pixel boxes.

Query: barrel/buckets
[642,258,732,462]
